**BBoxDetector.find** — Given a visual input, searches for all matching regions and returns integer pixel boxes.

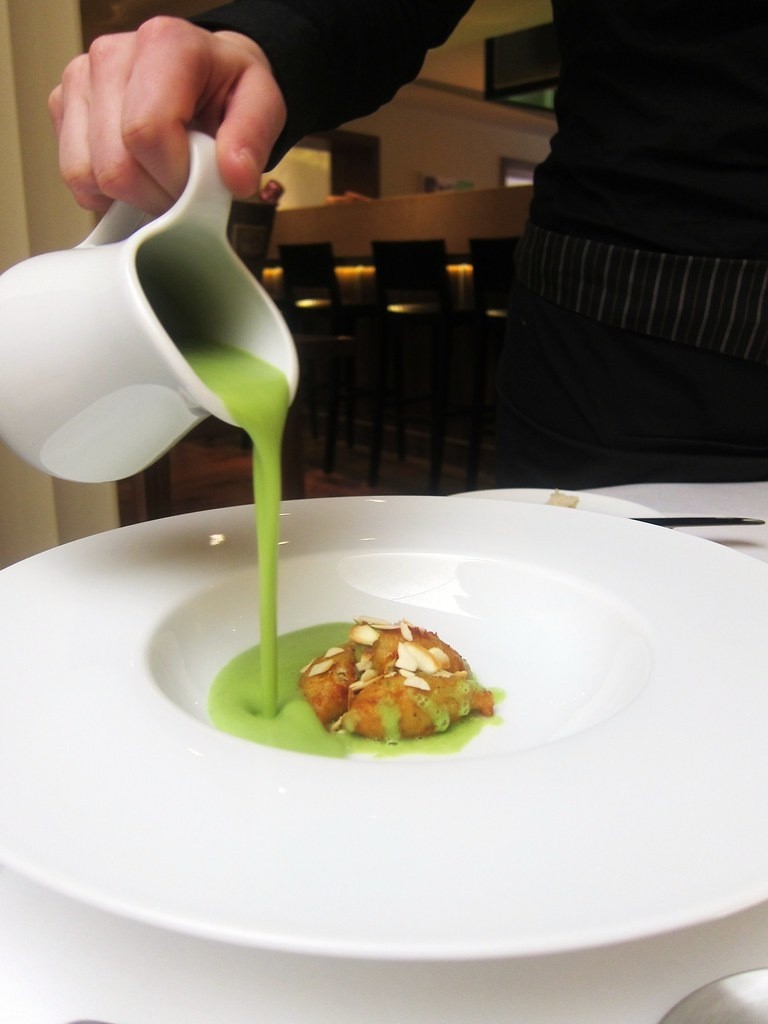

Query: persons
[44,0,768,483]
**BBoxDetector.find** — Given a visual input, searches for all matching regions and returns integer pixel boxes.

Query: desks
[267,185,537,259]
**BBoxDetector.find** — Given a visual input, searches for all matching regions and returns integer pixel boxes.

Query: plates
[453,489,671,529]
[0,495,768,962]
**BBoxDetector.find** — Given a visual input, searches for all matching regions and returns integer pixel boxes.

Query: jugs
[0,130,300,482]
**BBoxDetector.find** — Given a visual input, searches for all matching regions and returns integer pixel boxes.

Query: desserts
[299,617,494,739]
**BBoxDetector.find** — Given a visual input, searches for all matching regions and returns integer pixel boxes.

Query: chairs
[227,201,521,496]
